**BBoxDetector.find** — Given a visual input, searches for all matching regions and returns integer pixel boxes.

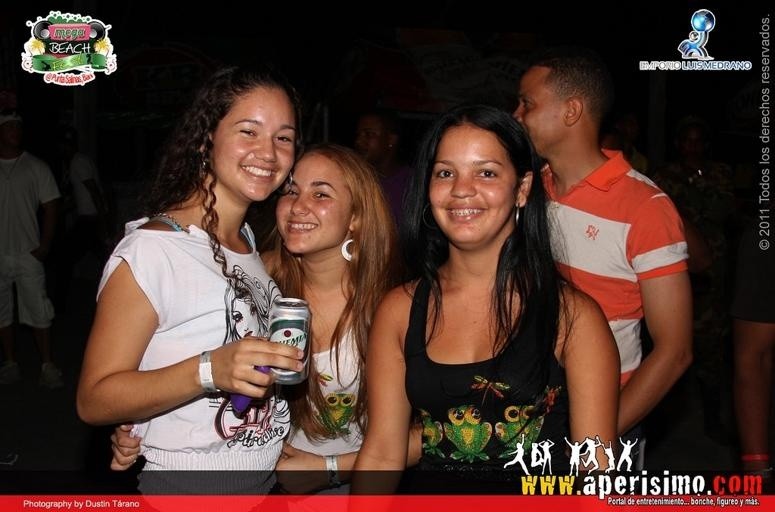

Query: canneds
[265,298,312,385]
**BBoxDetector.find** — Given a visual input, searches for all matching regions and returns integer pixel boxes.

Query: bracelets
[200,353,218,395]
[743,452,767,462]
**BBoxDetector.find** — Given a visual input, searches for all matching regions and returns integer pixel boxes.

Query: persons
[347,104,619,493]
[77,60,305,495]
[344,111,422,281]
[731,209,775,495]
[110,142,423,494]
[0,104,66,429]
[511,55,694,439]
[70,136,115,246]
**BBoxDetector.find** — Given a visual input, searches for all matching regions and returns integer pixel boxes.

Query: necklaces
[0,158,18,181]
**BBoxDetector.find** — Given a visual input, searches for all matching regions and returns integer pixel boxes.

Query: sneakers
[0,361,23,387]
[39,363,64,390]
